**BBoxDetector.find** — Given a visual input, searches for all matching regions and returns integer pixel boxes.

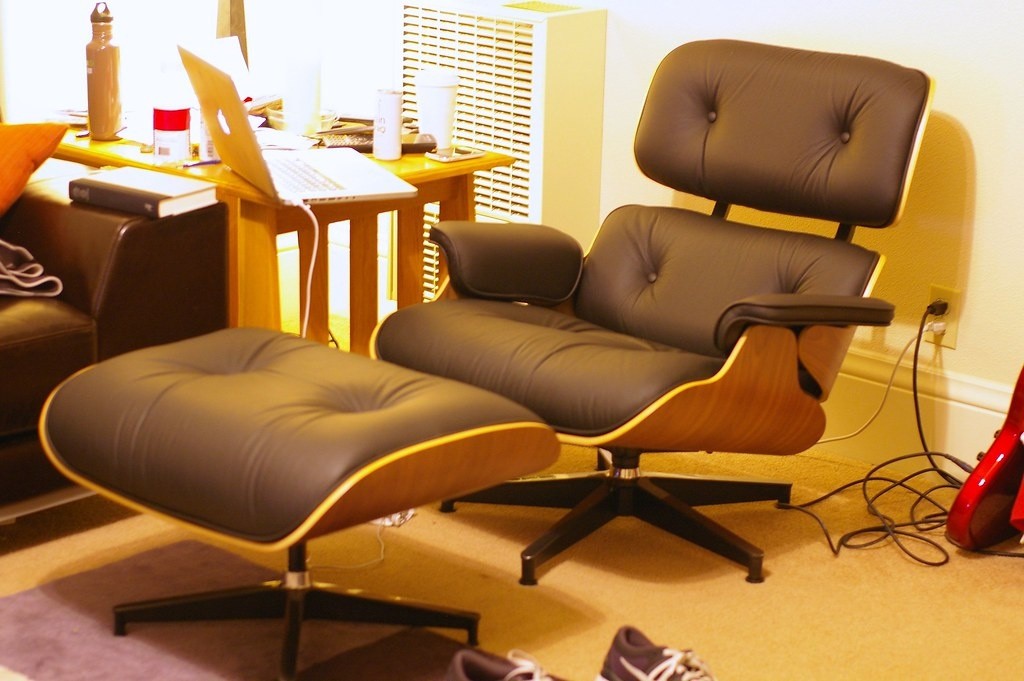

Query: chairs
[368,36,936,587]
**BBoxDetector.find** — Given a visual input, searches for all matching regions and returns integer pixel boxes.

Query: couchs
[0,116,228,505]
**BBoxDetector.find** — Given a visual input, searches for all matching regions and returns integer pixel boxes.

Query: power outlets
[924,282,961,349]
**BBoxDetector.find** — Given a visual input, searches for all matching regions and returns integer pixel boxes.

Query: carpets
[0,539,524,680]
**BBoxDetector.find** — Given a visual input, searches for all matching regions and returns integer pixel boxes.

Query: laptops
[176,44,418,205]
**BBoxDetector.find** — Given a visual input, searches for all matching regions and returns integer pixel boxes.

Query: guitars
[945,364,1024,550]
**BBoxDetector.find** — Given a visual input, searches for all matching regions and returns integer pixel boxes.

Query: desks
[54,118,518,357]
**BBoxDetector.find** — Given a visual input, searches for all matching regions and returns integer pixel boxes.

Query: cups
[152,105,191,165]
[372,89,402,160]
[416,69,458,159]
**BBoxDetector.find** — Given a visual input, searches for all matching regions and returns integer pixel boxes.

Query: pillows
[0,123,71,216]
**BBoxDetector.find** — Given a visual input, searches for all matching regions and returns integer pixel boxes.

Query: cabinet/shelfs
[389,0,607,303]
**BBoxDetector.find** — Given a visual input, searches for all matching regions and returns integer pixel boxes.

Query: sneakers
[444,649,566,681]
[596,626,716,681]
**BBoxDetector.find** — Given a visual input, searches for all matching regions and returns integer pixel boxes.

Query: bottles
[199,96,219,161]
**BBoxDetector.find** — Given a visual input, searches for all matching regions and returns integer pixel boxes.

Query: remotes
[323,134,447,158]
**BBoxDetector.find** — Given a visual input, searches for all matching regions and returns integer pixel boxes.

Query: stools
[38,321,561,681]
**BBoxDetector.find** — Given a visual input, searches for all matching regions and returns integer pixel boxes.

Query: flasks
[83,1,123,141]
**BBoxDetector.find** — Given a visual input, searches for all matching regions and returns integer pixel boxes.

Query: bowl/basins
[266,104,336,133]
